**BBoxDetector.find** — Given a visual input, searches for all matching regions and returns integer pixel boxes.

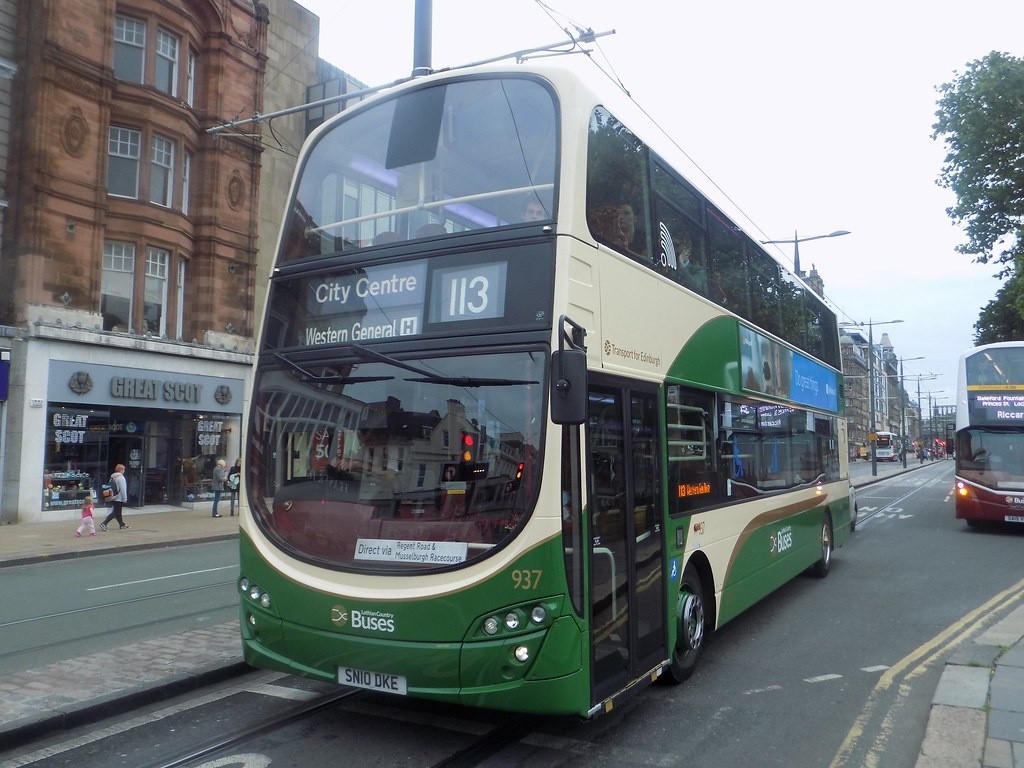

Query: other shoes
[75,531,81,537]
[231,512,234,516]
[213,514,223,518]
[90,533,97,537]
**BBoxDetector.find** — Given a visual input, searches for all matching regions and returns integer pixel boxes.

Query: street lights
[900,374,937,463]
[760,227,850,279]
[840,316,904,477]
[878,356,927,467]
[917,390,943,460]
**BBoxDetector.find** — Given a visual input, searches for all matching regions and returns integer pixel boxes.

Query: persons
[616,201,646,255]
[99,463,132,532]
[332,419,400,487]
[212,459,226,517]
[667,229,706,290]
[974,443,1001,463]
[76,496,96,536]
[228,456,241,515]
[520,197,549,223]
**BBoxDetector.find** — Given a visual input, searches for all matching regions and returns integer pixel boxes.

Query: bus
[236,53,858,726]
[874,431,899,462]
[955,341,1024,532]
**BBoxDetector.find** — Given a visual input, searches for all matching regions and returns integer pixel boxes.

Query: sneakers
[100,523,110,532]
[120,525,131,530]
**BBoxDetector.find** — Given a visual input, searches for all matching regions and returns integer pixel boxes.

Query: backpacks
[102,475,123,502]
